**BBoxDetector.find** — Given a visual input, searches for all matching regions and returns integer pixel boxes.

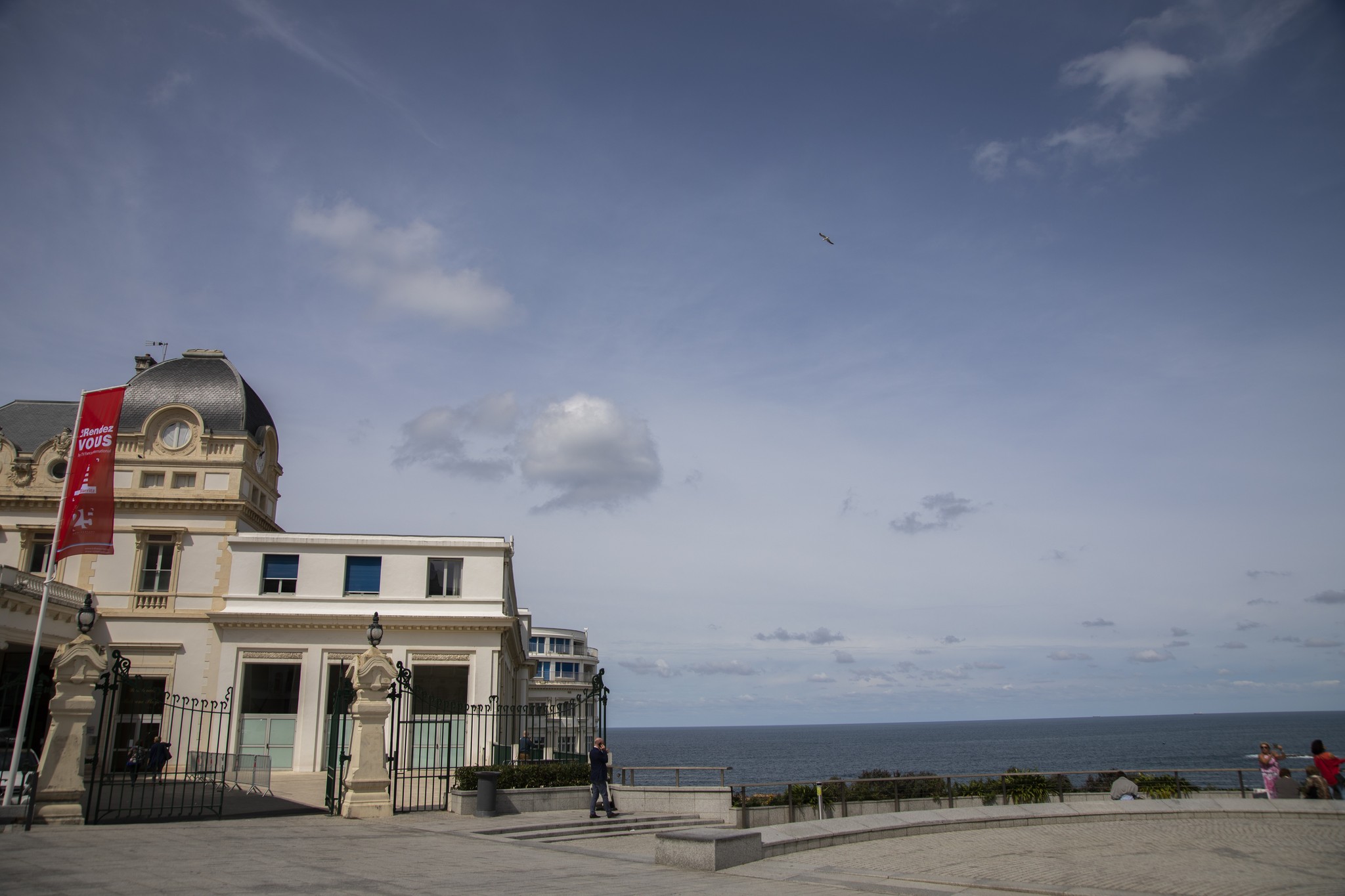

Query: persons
[1258,742,1286,799]
[1110,771,1139,800]
[148,736,172,786]
[1311,739,1345,800]
[520,730,535,765]
[1274,768,1300,798]
[1305,765,1332,799]
[127,739,146,787]
[589,738,620,819]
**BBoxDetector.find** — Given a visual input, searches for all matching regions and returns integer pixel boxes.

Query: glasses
[1260,746,1268,749]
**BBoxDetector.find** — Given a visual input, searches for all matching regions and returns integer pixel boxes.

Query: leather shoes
[607,812,620,818]
[590,814,600,818]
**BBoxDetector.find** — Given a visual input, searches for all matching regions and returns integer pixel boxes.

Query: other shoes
[150,784,155,786]
[158,779,162,785]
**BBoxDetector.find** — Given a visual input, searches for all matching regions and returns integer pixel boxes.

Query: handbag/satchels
[162,741,172,760]
[126,759,130,767]
[1334,773,1345,783]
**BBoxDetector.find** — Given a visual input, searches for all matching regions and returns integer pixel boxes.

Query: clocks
[254,435,269,477]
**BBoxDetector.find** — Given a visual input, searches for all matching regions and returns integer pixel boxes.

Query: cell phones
[598,742,603,748]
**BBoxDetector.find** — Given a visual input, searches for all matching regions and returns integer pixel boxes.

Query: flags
[55,386,126,563]
